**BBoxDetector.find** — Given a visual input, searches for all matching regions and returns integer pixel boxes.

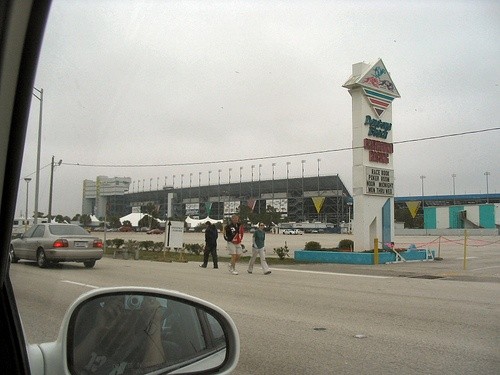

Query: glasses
[260,225,264,226]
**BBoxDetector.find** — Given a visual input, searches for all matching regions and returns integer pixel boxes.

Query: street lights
[23,177,32,231]
[451,174,457,205]
[420,175,426,209]
[484,170,491,203]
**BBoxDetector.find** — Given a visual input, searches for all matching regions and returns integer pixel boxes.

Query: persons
[199,220,219,269]
[223,213,244,276]
[72,295,170,375]
[247,221,272,276]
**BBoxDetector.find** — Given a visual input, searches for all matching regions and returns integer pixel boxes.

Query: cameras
[113,294,149,316]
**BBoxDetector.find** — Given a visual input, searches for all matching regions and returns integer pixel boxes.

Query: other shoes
[232,271,238,275]
[199,265,206,267]
[264,271,271,275]
[247,270,252,273]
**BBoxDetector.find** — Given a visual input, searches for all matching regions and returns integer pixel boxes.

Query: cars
[146,228,164,235]
[9,222,105,268]
[282,228,305,235]
